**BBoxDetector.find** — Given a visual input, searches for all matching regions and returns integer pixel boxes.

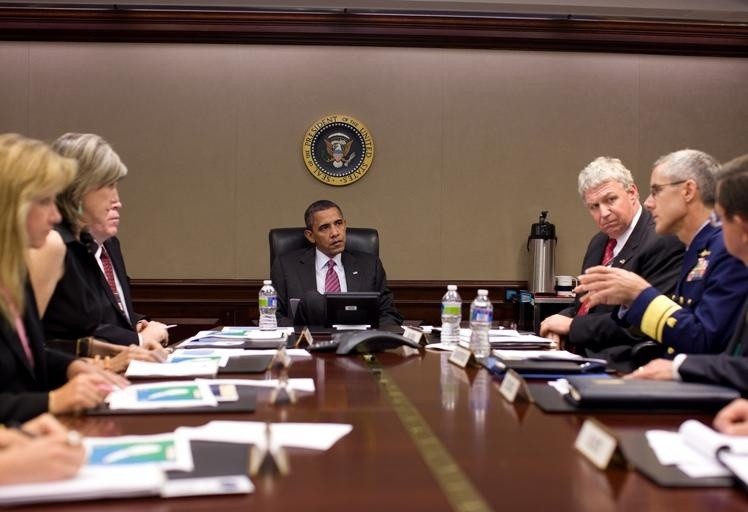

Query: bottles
[468,289,494,359]
[258,279,279,331]
[440,284,462,347]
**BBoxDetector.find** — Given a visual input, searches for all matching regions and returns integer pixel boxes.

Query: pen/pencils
[165,324,179,329]
[10,420,36,440]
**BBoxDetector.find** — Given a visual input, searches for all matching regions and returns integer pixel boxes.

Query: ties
[324,261,342,293]
[101,248,124,316]
[564,238,617,355]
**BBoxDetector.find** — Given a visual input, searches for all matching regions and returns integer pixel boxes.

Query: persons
[539,155,687,360]
[81,201,169,350]
[40,132,169,374]
[712,396,748,439]
[0,131,133,429]
[623,154,748,401]
[0,413,90,488]
[574,148,748,362]
[274,200,405,325]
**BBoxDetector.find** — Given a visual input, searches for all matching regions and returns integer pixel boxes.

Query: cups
[556,275,577,295]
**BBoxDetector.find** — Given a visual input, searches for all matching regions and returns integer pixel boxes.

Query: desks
[0,325,748,512]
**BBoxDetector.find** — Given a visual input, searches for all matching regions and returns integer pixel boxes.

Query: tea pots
[529,223,557,293]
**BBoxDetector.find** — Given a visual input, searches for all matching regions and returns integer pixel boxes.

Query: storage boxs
[516,293,577,337]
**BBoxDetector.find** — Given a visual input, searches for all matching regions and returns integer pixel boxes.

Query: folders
[494,358,583,373]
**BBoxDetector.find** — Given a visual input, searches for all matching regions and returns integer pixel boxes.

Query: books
[493,349,583,375]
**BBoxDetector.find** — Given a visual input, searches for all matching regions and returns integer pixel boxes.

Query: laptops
[324,291,381,330]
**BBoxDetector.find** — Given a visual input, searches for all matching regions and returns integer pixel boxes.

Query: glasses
[650,179,699,196]
[709,210,726,227]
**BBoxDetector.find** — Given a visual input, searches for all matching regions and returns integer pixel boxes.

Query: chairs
[268,227,380,272]
